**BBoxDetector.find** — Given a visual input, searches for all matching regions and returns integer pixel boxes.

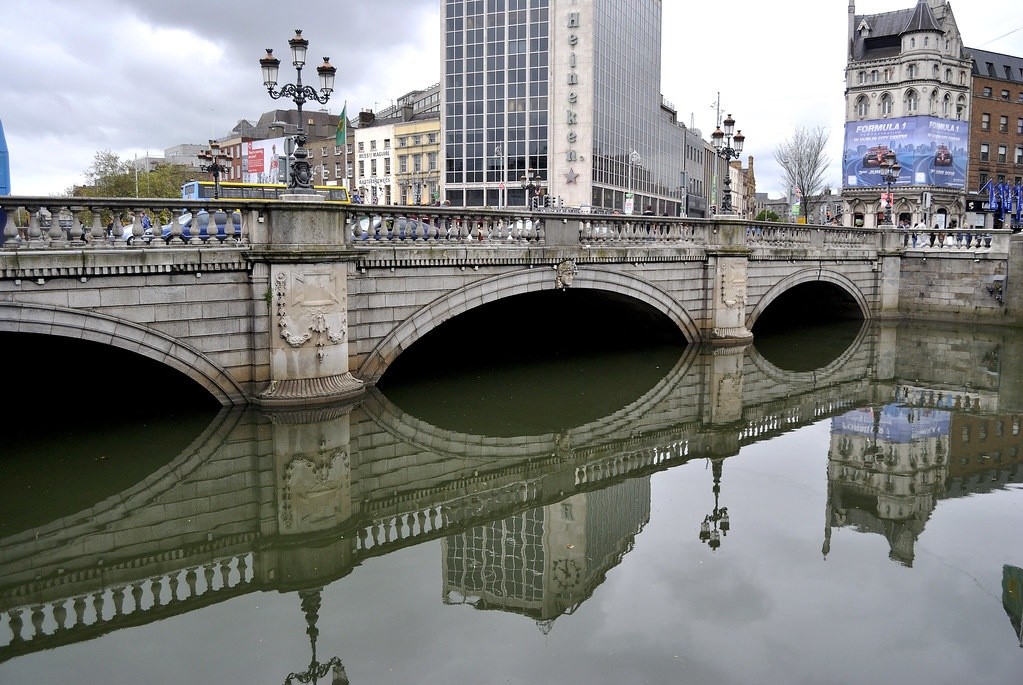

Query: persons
[612,210,622,233]
[660,213,672,241]
[897,220,911,250]
[642,206,656,242]
[130,211,152,231]
[912,219,927,249]
[947,222,974,249]
[79,221,88,244]
[929,222,939,247]
[107,219,114,235]
[761,218,775,243]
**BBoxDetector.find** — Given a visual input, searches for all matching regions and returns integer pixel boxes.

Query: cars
[351,216,447,240]
[140,209,244,244]
[110,220,157,247]
[504,219,584,240]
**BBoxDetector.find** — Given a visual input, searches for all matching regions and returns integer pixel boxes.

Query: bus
[180,182,352,207]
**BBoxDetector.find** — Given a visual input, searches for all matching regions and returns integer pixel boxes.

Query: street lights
[259,29,337,203]
[197,139,233,199]
[877,165,897,229]
[283,589,351,685]
[698,460,729,553]
[520,168,542,211]
[712,114,744,219]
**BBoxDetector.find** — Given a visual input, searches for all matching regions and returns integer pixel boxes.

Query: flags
[986,180,1023,223]
[335,106,345,146]
